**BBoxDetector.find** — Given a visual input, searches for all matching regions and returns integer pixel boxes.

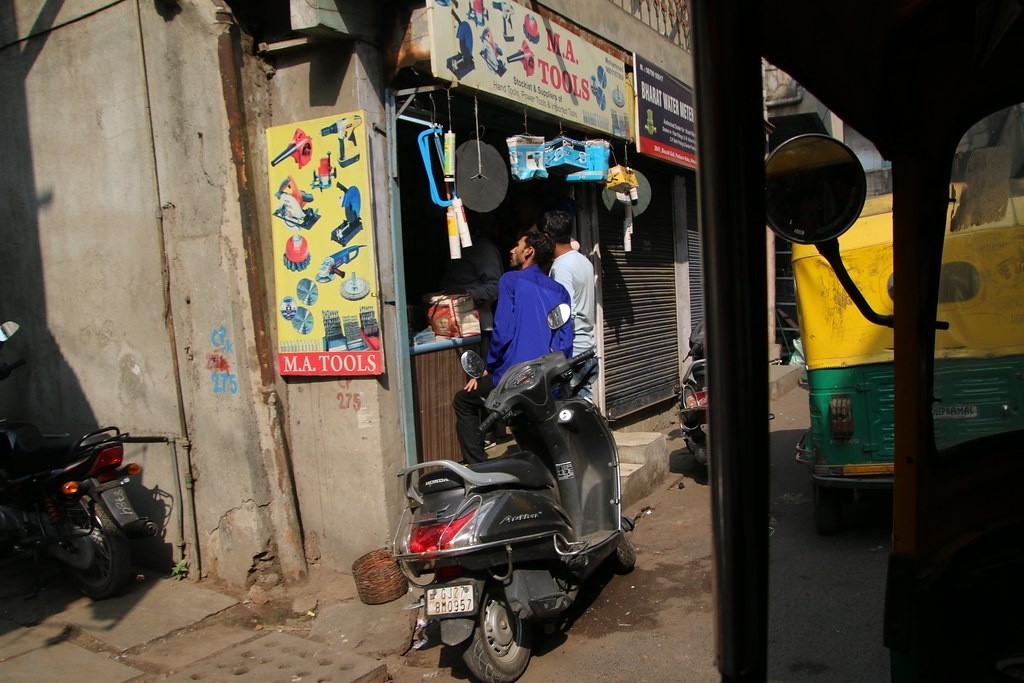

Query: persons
[544,212,599,408]
[455,232,574,466]
[416,232,504,358]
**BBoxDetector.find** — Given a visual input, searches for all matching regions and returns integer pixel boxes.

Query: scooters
[391,302,638,682]
[678,320,708,484]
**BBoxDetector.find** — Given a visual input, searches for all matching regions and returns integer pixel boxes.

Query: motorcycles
[0,321,159,600]
[791,177,1024,536]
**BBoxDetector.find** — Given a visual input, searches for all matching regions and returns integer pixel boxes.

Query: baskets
[351,548,408,606]
